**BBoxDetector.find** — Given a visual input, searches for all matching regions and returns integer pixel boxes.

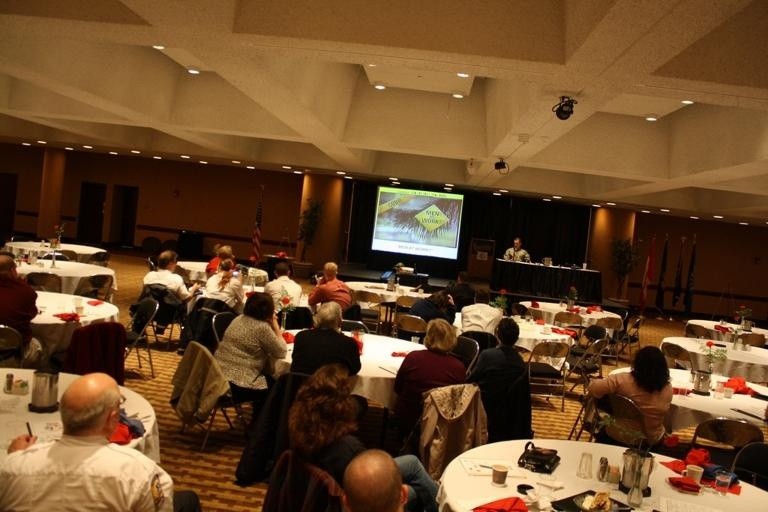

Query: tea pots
[619,438,654,497]
[691,369,712,396]
[29,367,60,414]
[743,318,755,332]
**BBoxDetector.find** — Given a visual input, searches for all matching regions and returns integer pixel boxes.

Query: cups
[411,336,420,345]
[419,289,423,294]
[492,465,509,484]
[512,315,552,335]
[576,452,594,481]
[682,464,704,486]
[542,257,552,266]
[678,383,733,401]
[76,307,84,316]
[714,470,732,497]
[582,263,587,269]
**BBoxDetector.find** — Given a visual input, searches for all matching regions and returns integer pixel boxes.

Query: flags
[639,239,657,311]
[249,191,264,268]
[655,239,669,311]
[682,243,697,311]
[671,241,685,309]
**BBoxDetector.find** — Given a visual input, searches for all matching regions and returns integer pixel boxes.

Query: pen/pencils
[480,464,492,469]
[27,423,33,437]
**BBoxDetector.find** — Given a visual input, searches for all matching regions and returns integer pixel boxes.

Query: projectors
[399,266,414,274]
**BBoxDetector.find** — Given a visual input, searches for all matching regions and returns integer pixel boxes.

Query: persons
[397,290,457,345]
[448,270,474,313]
[0,372,175,512]
[288,361,439,512]
[212,292,288,436]
[142,250,201,352]
[1,254,44,370]
[588,344,673,452]
[502,236,532,263]
[205,245,237,279]
[392,317,465,421]
[205,258,244,309]
[466,317,533,411]
[263,263,303,328]
[340,448,409,512]
[290,301,362,377]
[308,261,352,317]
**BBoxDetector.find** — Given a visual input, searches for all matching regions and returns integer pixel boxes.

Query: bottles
[598,457,611,483]
[719,319,727,325]
[627,469,643,507]
[6,374,14,392]
[733,330,750,352]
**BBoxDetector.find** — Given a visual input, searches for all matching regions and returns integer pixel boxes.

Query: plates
[551,490,636,512]
[573,493,618,512]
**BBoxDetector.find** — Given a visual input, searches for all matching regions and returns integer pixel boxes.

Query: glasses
[107,393,128,422]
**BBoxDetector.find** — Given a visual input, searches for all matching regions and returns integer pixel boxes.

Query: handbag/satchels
[519,441,561,474]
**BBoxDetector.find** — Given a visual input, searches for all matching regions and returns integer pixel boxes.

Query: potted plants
[290,198,325,277]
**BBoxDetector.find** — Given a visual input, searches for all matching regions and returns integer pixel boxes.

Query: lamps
[553,95,578,120]
[495,160,510,176]
